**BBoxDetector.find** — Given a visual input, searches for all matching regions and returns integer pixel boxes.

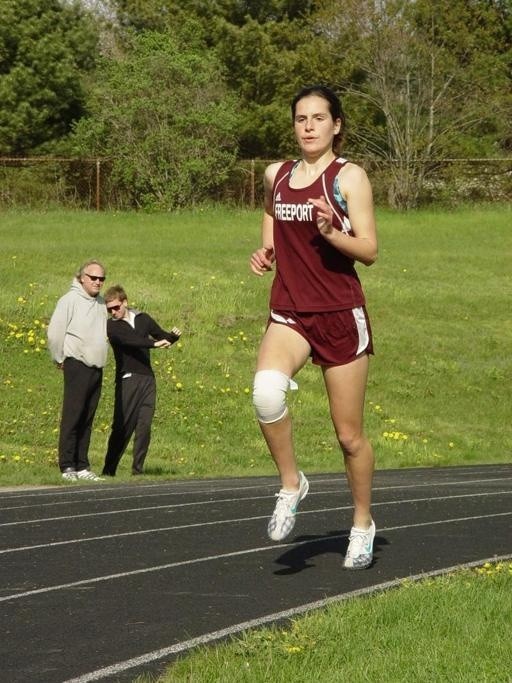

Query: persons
[248,84,379,571]
[47,261,108,481]
[104,284,182,476]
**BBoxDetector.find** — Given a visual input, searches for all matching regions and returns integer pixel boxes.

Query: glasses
[107,303,123,313]
[85,274,106,282]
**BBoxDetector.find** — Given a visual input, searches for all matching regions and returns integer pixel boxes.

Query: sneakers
[267,470,310,541]
[342,519,376,571]
[77,466,107,482]
[62,467,79,482]
